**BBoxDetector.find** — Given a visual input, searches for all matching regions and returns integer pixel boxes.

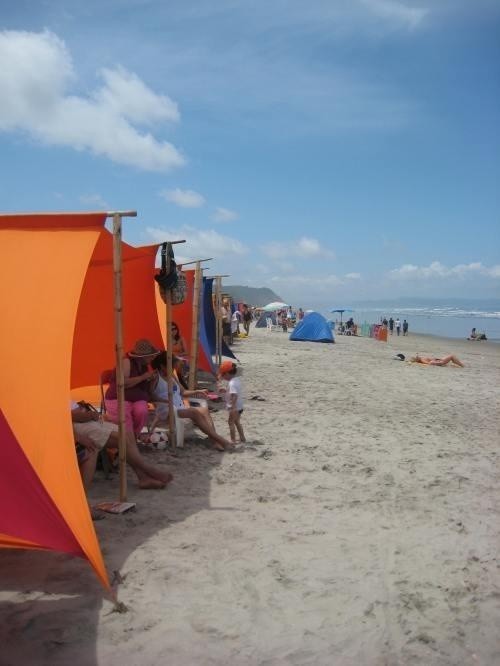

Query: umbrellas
[328,303,355,326]
[262,301,289,330]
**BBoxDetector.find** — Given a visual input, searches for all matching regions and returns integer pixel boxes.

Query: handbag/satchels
[156,242,188,305]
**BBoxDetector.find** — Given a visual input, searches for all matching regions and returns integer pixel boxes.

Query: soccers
[148,432,170,450]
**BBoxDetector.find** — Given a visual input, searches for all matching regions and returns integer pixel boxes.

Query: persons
[69,398,173,490]
[378,314,408,336]
[102,339,159,440]
[471,327,482,340]
[410,353,465,367]
[266,312,272,321]
[149,351,235,452]
[217,360,247,442]
[170,321,187,357]
[336,322,346,335]
[278,305,305,332]
[347,317,358,336]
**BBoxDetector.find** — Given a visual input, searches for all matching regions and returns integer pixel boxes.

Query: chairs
[343,324,353,336]
[99,368,113,414]
[266,318,279,333]
[147,397,209,448]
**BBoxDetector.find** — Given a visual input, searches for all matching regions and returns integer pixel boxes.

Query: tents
[288,311,336,343]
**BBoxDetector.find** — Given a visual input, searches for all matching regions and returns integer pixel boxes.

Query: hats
[219,361,234,372]
[128,339,160,357]
